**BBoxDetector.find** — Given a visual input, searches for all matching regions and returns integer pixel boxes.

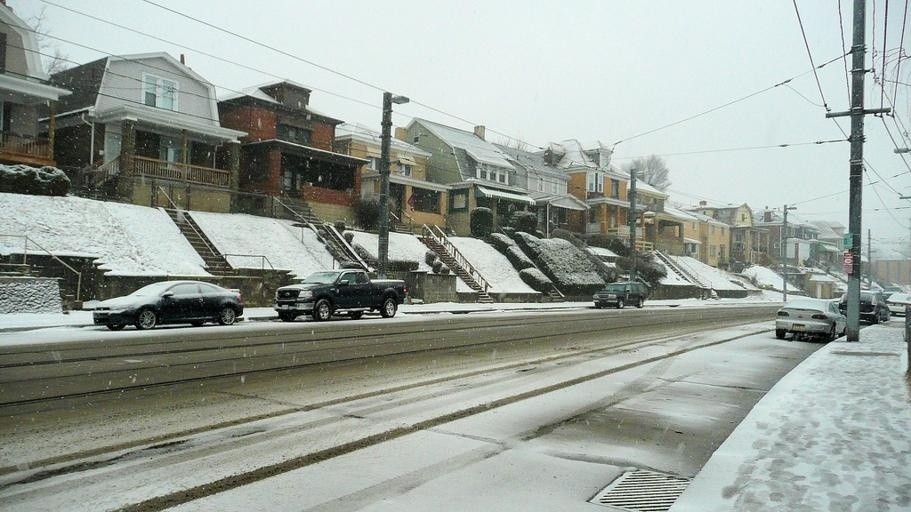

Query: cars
[881,284,911,317]
[593,282,650,311]
[90,279,245,331]
[775,298,847,341]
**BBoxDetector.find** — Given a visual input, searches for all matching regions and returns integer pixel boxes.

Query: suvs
[837,289,891,323]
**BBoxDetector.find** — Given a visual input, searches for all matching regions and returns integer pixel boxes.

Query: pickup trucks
[273,269,410,321]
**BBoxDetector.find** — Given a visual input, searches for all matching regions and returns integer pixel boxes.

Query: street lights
[629,168,645,281]
[892,146,911,154]
[372,89,411,280]
[780,202,796,301]
